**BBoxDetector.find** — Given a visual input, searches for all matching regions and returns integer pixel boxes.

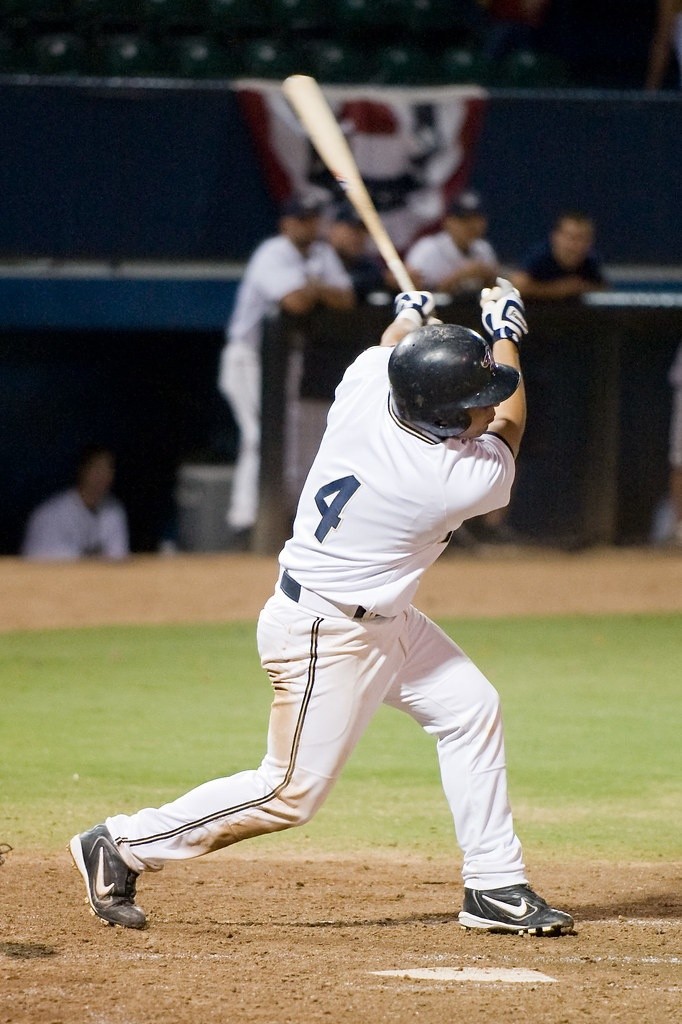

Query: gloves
[480,277,529,349]
[395,289,435,324]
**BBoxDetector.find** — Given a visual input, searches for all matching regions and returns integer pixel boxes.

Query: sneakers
[458,884,574,938]
[68,823,146,929]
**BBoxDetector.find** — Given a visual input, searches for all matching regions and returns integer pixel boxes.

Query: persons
[215,0,682,551]
[20,438,131,559]
[67,279,577,936]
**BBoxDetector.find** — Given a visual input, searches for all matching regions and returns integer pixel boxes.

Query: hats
[281,198,324,224]
[447,190,489,219]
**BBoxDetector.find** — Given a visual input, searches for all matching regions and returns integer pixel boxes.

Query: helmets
[388,325,520,437]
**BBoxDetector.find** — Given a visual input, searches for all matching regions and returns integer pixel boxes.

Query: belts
[280,571,381,619]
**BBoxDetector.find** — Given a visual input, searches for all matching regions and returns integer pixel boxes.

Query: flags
[242,60,484,264]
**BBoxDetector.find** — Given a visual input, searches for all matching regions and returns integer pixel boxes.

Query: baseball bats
[280,72,431,331]
